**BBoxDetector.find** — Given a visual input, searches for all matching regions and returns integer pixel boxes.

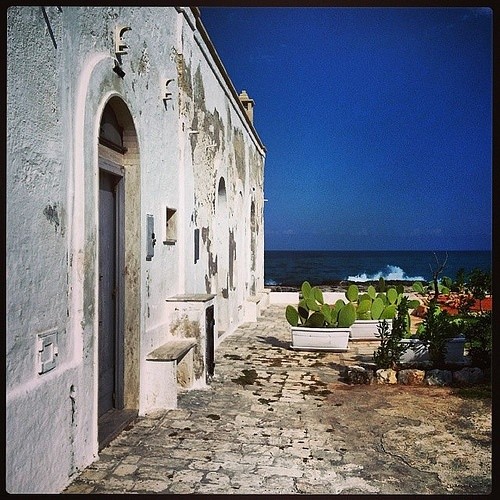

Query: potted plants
[374,251,472,372]
[345,283,413,339]
[285,280,355,352]
